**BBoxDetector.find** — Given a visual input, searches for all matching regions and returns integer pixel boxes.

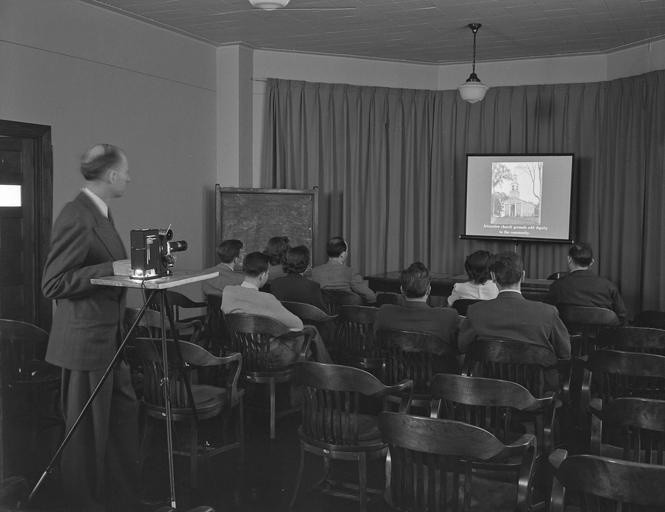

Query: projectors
[129,225,187,280]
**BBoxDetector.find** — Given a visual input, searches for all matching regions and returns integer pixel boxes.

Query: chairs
[0,318,69,452]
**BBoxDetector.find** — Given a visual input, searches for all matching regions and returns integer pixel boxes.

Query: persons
[199,240,246,298]
[546,241,628,321]
[446,250,503,307]
[41,143,171,510]
[262,236,290,286]
[270,246,350,364]
[461,252,573,434]
[303,236,377,304]
[218,253,337,409]
[367,262,458,383]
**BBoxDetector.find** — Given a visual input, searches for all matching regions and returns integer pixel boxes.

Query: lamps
[455,22,490,105]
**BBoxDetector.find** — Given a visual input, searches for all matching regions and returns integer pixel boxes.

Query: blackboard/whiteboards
[215,188,319,274]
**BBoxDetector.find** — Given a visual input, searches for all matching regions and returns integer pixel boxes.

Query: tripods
[15,291,210,512]
